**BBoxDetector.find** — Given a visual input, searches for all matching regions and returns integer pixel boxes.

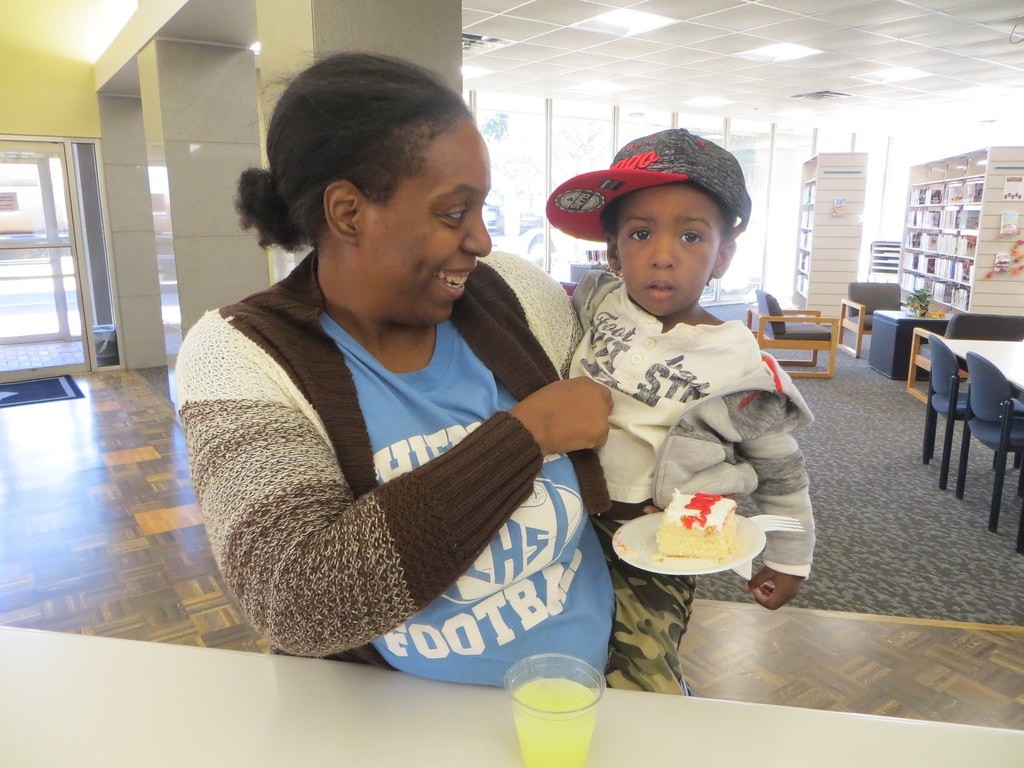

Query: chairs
[922,334,1024,489]
[836,282,909,358]
[746,308,839,378]
[955,351,1024,533]
[906,313,1024,406]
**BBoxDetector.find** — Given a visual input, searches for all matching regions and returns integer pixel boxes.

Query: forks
[747,514,805,534]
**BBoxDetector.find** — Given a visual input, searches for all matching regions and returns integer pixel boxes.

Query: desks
[941,340,1024,394]
[867,310,954,381]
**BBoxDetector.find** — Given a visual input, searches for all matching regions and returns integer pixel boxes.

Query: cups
[504,653,606,768]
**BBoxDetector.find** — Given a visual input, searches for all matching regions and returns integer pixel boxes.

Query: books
[902,181,983,312]
[796,183,812,296]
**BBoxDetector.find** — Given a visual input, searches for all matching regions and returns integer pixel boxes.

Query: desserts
[655,488,737,563]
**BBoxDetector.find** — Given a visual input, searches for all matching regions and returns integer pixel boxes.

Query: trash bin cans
[91,323,119,367]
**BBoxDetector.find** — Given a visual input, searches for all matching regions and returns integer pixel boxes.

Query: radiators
[868,241,901,274]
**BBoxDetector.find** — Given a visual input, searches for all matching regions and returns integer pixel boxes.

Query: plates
[610,511,767,576]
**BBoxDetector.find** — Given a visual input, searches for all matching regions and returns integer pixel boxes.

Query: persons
[170,50,737,690]
[547,128,816,696]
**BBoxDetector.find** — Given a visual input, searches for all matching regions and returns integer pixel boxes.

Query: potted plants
[906,288,933,317]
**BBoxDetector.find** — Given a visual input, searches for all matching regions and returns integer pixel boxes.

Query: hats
[546,128,752,243]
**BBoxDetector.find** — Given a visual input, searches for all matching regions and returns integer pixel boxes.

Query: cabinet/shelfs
[790,152,868,325]
[896,144,1023,316]
